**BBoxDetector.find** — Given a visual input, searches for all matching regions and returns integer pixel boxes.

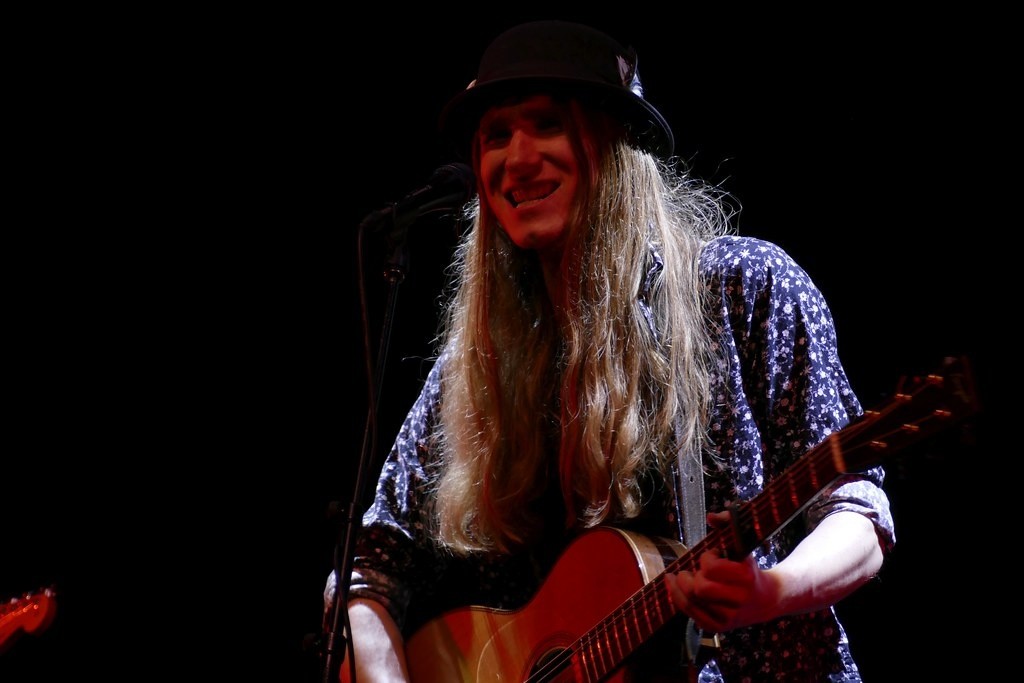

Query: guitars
[326,351,982,682]
[0,582,59,654]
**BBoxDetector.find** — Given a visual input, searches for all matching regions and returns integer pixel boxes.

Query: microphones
[366,162,476,228]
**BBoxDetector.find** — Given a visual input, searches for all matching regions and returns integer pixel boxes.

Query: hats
[440,21,674,168]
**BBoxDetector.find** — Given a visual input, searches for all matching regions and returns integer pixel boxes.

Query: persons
[321,20,896,683]
